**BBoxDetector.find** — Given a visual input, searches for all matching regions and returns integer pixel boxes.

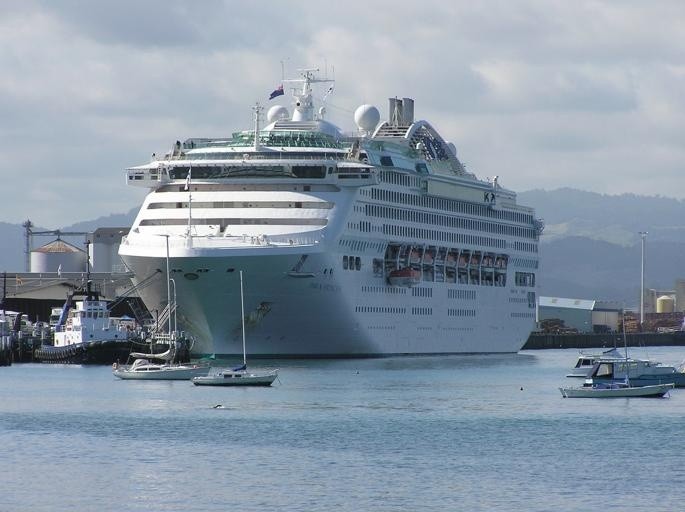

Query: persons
[191,141,193,148]
[484,193,489,202]
[491,193,495,203]
[173,331,190,365]
[492,175,499,191]
[176,140,180,149]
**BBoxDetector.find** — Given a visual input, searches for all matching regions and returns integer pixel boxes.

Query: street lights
[637,231,649,332]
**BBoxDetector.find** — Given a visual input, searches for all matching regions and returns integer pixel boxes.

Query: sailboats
[33,232,279,387]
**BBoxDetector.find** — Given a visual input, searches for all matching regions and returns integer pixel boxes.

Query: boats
[558,314,684,398]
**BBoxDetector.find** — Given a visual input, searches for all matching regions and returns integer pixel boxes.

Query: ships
[117,55,544,357]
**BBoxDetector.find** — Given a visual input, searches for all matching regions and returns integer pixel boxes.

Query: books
[268,84,284,100]
